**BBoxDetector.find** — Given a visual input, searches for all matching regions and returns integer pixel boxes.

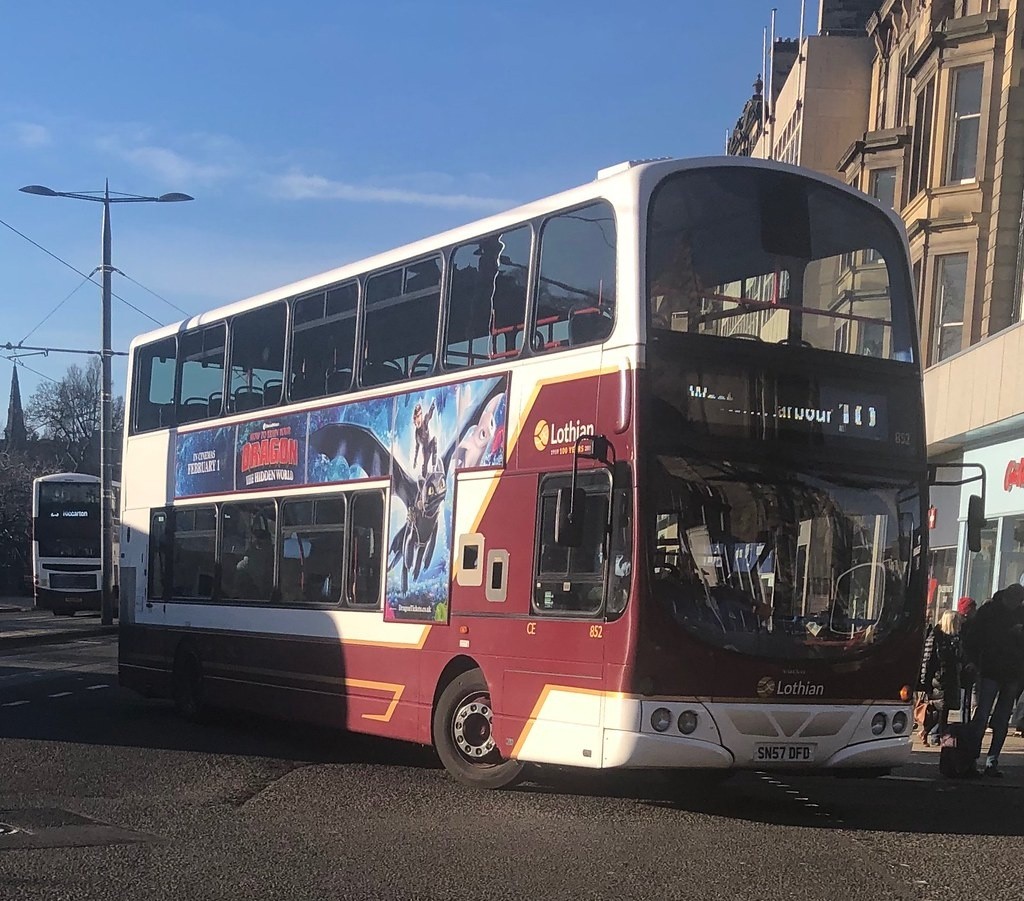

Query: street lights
[17,178,198,627]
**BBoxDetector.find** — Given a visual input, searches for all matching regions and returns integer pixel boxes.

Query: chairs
[158,301,813,430]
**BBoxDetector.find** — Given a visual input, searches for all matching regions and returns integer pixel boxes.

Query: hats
[958,598,976,616]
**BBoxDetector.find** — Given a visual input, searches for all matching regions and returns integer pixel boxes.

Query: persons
[234,528,273,600]
[913,597,1024,747]
[964,584,1024,777]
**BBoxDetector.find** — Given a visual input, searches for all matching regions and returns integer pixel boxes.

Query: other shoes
[985,766,1002,777]
[931,739,940,746]
[917,730,930,747]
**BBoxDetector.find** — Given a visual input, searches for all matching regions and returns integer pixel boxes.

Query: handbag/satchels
[939,746,977,778]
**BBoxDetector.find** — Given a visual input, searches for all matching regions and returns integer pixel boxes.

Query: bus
[31,471,123,618]
[117,155,992,796]
